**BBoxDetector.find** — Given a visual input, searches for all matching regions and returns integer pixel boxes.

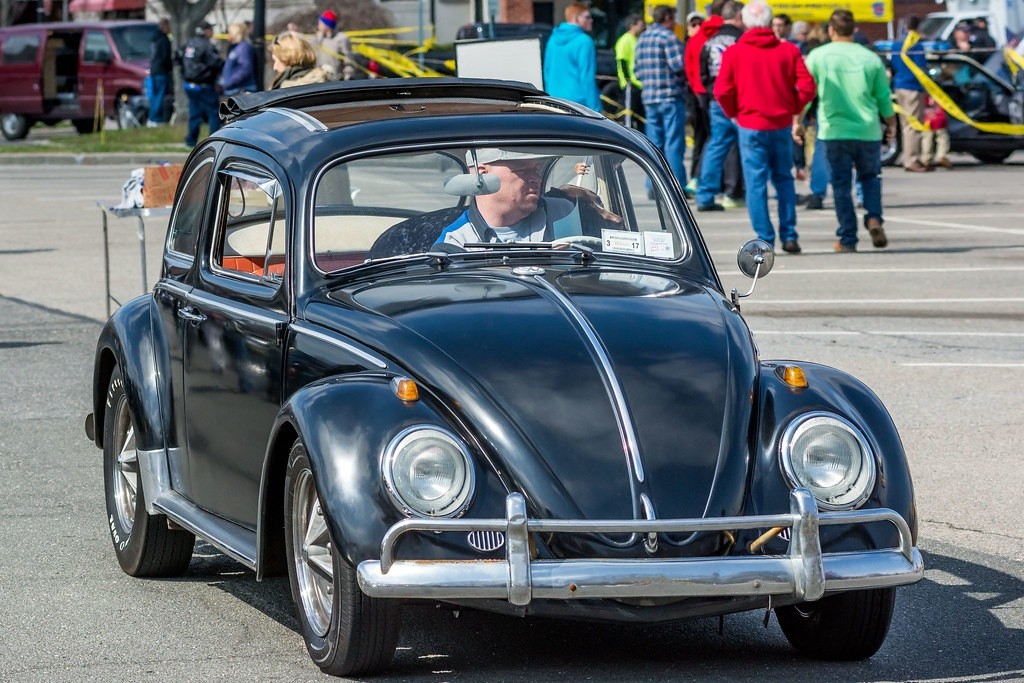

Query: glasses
[689,20,702,28]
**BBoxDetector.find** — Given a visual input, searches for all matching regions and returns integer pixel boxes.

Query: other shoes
[723,195,747,208]
[686,177,700,193]
[834,241,856,252]
[808,196,824,209]
[906,159,926,171]
[868,218,887,247]
[936,158,952,168]
[697,201,724,211]
[784,241,801,252]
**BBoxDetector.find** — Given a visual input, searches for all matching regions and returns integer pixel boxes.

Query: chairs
[251,260,366,275]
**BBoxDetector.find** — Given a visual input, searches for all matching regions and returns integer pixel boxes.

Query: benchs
[223,251,368,279]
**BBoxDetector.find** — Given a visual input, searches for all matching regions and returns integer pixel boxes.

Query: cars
[86,77,925,677]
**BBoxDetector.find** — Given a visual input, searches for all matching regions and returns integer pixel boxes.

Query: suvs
[859,11,1024,169]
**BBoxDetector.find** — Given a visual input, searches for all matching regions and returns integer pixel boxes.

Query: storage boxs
[143,165,183,207]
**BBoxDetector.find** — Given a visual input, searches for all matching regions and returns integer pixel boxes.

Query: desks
[96,200,176,316]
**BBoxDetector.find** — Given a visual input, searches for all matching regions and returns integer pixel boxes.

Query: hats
[466,143,563,167]
[197,20,218,30]
[320,10,339,29]
[687,10,704,24]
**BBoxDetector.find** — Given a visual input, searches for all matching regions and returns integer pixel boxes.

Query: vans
[0,19,177,144]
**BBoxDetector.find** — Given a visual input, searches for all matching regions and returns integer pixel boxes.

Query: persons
[789,7,897,253]
[430,147,625,254]
[543,3,603,114]
[613,14,645,135]
[308,10,356,82]
[632,4,690,199]
[146,19,173,128]
[216,21,257,99]
[684,0,997,209]
[175,21,224,147]
[713,0,817,254]
[268,34,326,91]
